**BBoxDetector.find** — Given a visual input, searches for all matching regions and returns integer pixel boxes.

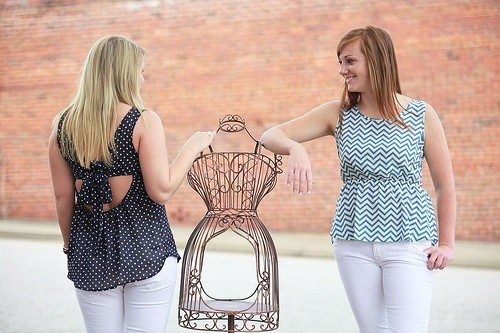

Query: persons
[48,35,215,333]
[260,25,457,331]
